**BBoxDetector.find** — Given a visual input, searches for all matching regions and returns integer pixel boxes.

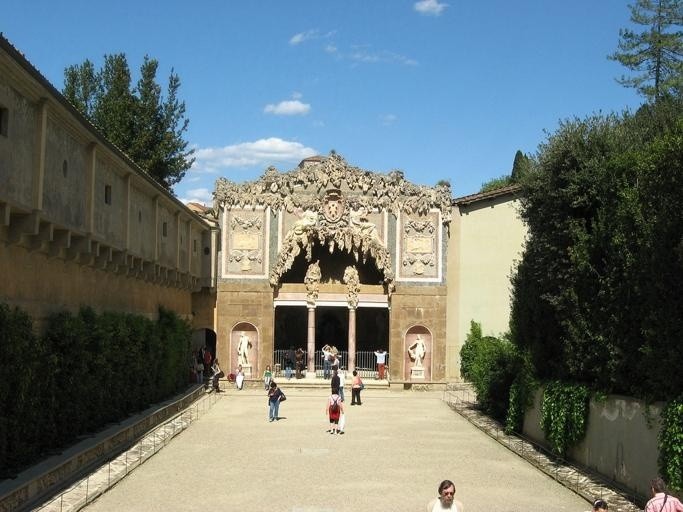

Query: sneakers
[269,417,278,422]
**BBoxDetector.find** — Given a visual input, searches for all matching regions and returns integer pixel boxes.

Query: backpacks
[330,393,340,414]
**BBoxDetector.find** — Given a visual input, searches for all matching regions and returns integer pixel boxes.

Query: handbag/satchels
[359,384,364,390]
[197,363,205,371]
[280,393,286,401]
[216,371,224,378]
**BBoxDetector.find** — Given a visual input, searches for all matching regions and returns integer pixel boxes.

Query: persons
[235,330,253,364]
[347,370,363,405]
[324,386,344,435]
[642,476,682,512]
[426,480,462,512]
[407,334,426,367]
[590,498,608,512]
[189,344,340,395]
[371,348,387,380]
[336,368,345,403]
[267,381,282,422]
